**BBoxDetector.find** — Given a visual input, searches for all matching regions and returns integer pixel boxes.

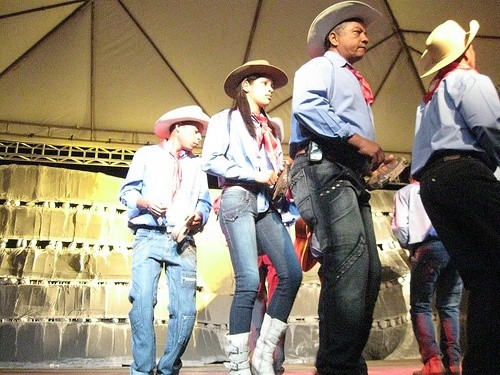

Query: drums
[368,157,407,189]
[171,212,193,244]
[271,166,289,201]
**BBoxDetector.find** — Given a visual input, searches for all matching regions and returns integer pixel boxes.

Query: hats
[419,19,481,78]
[224,59,288,99]
[307,1,383,59]
[153,105,210,139]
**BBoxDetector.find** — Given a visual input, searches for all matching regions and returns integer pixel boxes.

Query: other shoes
[412,360,462,375]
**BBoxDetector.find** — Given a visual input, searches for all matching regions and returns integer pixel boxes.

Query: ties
[345,62,374,104]
[422,62,481,104]
[172,150,191,197]
[249,111,281,170]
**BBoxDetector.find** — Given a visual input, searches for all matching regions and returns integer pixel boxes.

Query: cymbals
[269,162,292,203]
[170,210,196,244]
[365,153,412,191]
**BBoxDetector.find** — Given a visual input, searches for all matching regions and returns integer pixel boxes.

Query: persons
[200,60,303,375]
[288,2,393,375]
[392,162,462,375]
[409,20,500,375]
[118,105,213,375]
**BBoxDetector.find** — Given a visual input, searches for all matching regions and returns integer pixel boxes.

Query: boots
[250,312,288,375]
[223,332,252,375]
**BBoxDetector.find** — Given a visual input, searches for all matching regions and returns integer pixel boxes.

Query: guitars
[291,215,319,273]
